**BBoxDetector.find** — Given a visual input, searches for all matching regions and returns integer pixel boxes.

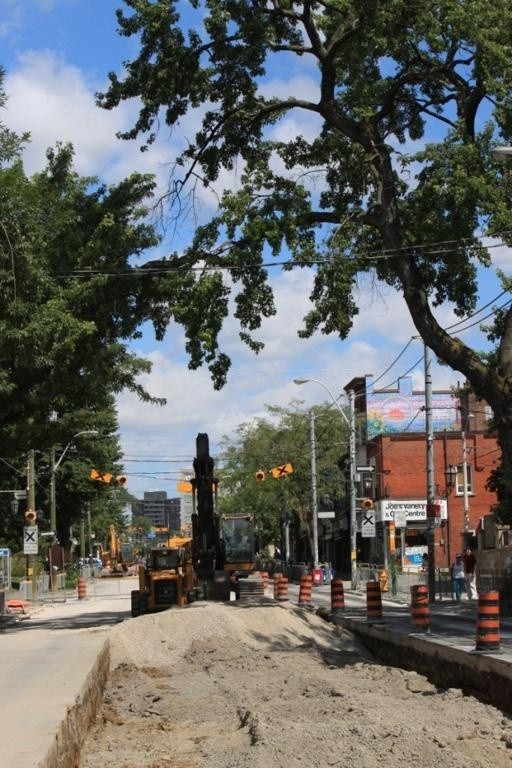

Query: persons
[451,553,465,601]
[44,555,55,590]
[421,553,430,586]
[274,549,279,559]
[463,548,480,600]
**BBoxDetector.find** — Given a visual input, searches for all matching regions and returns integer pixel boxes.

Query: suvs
[78,557,101,570]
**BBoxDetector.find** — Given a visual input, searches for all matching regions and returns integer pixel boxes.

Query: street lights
[490,145,512,161]
[86,488,121,574]
[291,376,360,590]
[47,428,98,546]
[441,462,459,571]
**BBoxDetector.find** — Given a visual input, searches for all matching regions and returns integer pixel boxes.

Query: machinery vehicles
[131,430,267,613]
[87,516,193,578]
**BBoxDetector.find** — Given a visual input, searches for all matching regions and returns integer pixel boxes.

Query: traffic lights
[254,469,266,484]
[359,497,374,509]
[23,509,37,523]
[115,473,128,486]
